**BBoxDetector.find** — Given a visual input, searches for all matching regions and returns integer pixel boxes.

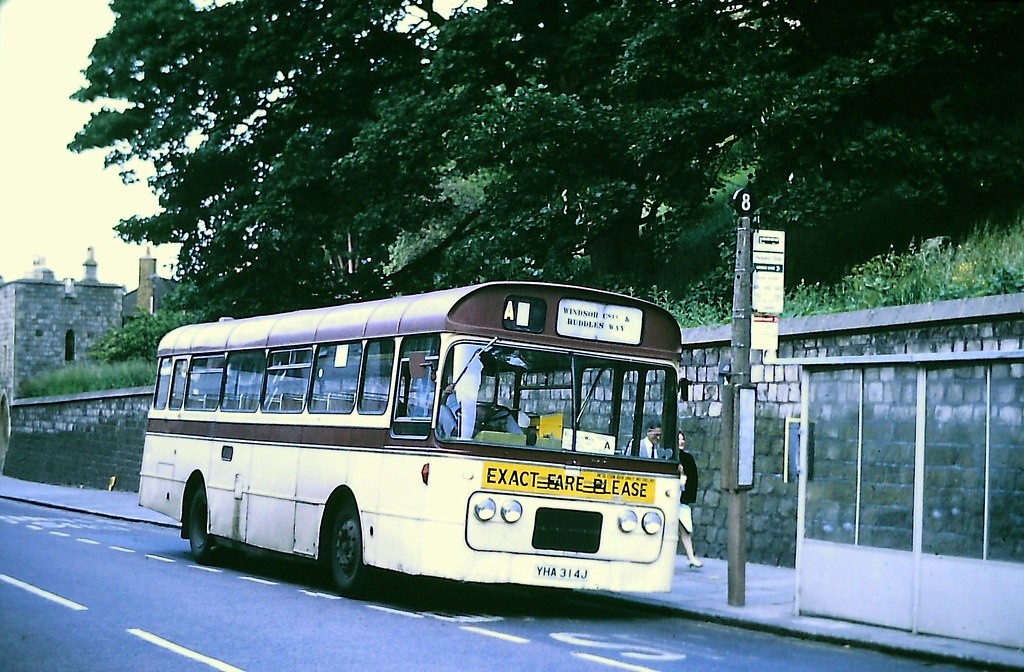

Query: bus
[140,281,693,603]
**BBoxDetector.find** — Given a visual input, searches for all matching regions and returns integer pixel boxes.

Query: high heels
[687,557,703,568]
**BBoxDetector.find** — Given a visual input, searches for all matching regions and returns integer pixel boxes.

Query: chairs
[161,391,435,420]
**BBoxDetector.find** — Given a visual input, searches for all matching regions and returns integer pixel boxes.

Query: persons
[677,431,704,569]
[437,379,525,441]
[627,419,663,458]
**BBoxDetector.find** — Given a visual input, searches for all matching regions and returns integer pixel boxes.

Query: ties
[651,444,655,459]
